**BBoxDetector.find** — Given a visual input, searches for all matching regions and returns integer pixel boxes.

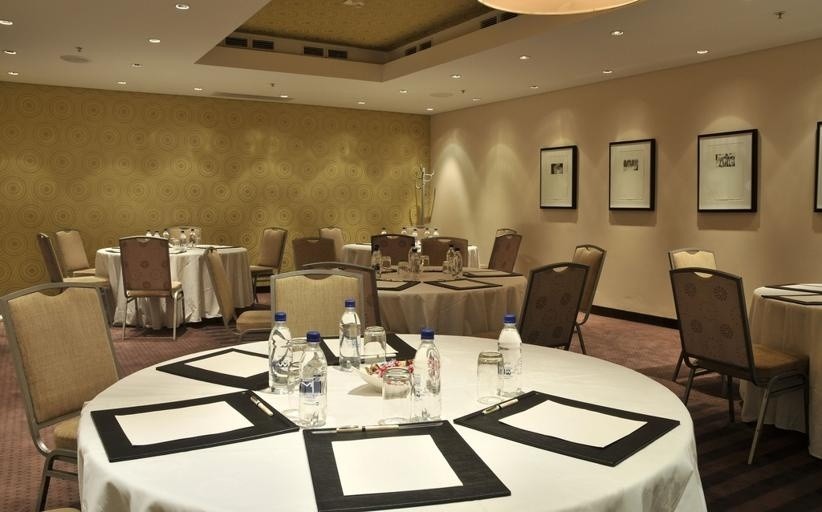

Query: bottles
[299,330,326,426]
[497,315,523,397]
[337,299,364,371]
[412,330,439,420]
[379,225,440,238]
[269,311,294,394]
[145,228,197,253]
[370,245,463,279]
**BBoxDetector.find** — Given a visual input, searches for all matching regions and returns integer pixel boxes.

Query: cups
[384,366,411,421]
[365,324,386,368]
[476,351,505,405]
[291,337,305,378]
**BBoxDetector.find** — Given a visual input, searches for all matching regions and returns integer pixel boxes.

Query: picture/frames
[696,129,757,212]
[608,138,655,210]
[539,144,576,209]
[812,121,822,212]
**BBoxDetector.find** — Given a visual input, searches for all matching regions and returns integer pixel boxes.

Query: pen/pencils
[336,425,400,433]
[482,398,518,415]
[250,395,273,416]
[360,354,397,359]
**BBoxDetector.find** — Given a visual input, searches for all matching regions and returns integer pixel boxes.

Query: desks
[95,243,253,324]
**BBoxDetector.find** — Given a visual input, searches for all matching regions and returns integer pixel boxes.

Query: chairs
[1,276,129,512]
[668,267,812,466]
[167,226,201,245]
[118,235,187,341]
[56,229,96,277]
[38,233,115,325]
[665,245,719,386]
[248,227,288,310]
[291,225,608,357]
